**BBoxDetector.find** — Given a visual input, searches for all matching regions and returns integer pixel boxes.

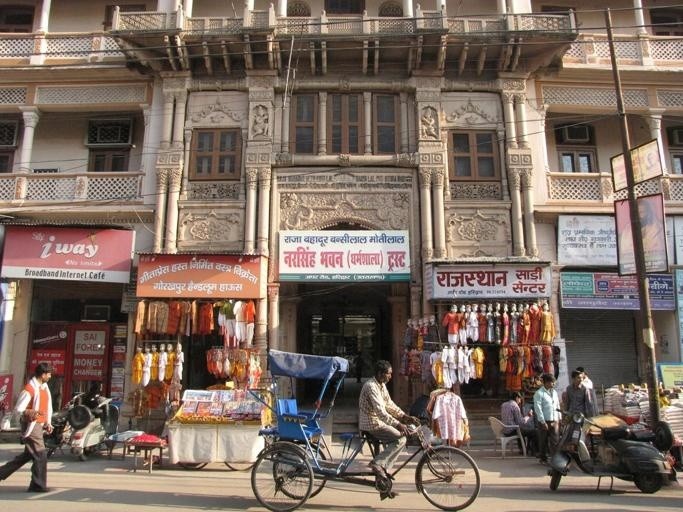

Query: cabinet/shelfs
[109,441,142,458]
[129,443,165,474]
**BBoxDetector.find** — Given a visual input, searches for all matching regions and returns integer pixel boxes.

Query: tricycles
[246,348,481,512]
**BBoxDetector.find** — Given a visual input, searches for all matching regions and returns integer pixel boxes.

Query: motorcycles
[46,392,86,459]
[70,395,121,462]
[546,408,673,496]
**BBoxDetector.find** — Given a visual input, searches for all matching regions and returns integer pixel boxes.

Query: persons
[500,390,538,456]
[0,362,53,492]
[533,373,561,465]
[131,342,184,384]
[356,358,420,480]
[567,370,594,425]
[404,302,554,350]
[574,367,599,415]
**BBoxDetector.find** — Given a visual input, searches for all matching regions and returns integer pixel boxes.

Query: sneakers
[380,491,398,500]
[369,460,389,481]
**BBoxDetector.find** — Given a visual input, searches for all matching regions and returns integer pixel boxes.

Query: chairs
[487,416,528,458]
[276,398,322,443]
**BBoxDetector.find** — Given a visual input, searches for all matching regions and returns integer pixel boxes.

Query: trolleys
[166,420,265,472]
